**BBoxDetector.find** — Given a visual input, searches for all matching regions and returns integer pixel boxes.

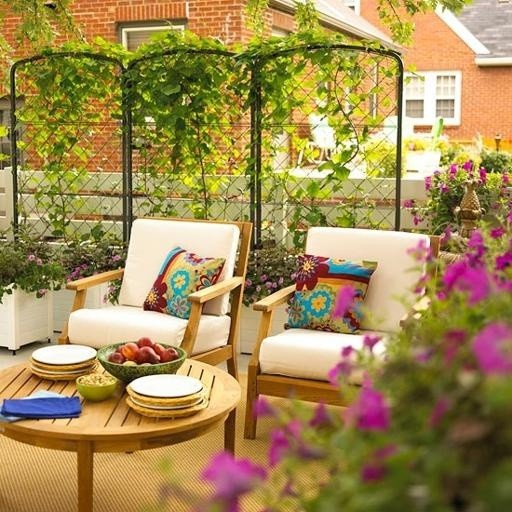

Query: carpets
[0,372,348,512]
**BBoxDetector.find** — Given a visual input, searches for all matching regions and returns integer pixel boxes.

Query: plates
[29,340,99,384]
[125,371,211,419]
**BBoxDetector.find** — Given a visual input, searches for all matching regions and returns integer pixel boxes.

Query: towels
[0,396,81,419]
[1,390,66,422]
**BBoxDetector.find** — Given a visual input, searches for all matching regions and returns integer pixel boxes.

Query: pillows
[144,246,226,320]
[285,255,377,334]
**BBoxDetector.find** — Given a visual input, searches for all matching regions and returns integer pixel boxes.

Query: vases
[237,302,290,354]
[1,279,54,355]
[54,281,117,332]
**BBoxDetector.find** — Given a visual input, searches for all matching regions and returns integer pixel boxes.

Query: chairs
[245,227,440,439]
[58,216,253,383]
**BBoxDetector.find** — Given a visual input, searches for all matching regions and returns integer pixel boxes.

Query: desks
[0,359,240,512]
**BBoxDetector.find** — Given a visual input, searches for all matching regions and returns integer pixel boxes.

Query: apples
[109,337,179,367]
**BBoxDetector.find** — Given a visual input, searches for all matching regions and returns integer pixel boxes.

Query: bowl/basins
[96,339,188,384]
[75,373,119,403]
